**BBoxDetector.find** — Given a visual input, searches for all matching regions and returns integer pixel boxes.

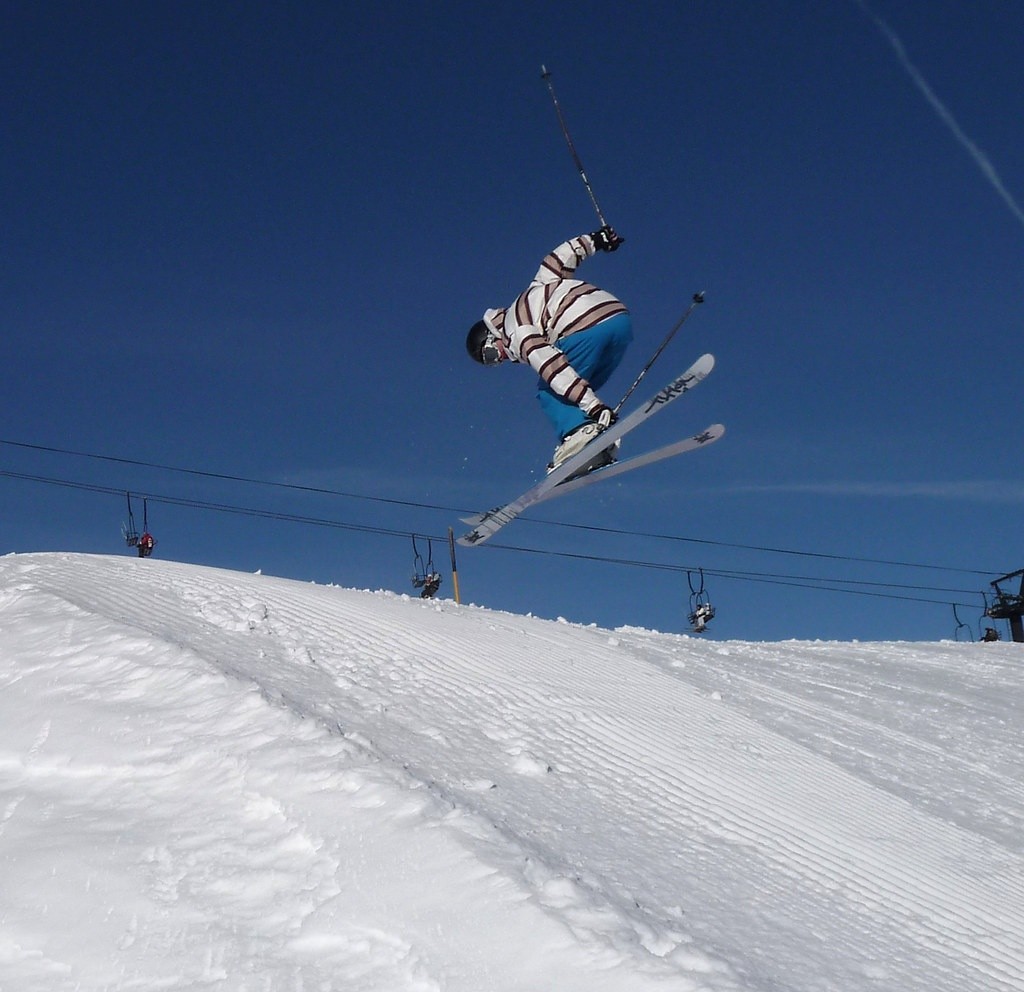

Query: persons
[465,225,633,483]
[138,532,153,557]
[694,602,712,633]
[424,572,441,598]
[979,628,998,642]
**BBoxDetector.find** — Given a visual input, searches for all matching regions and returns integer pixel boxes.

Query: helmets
[466,320,490,363]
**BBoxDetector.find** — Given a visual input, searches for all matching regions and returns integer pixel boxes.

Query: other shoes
[551,424,602,462]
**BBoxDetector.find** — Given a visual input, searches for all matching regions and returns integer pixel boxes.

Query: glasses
[482,340,502,366]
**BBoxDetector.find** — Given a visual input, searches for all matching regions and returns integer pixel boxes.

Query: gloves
[590,403,614,427]
[591,226,624,251]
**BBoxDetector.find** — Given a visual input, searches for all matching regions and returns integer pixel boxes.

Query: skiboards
[456,351,725,546]
